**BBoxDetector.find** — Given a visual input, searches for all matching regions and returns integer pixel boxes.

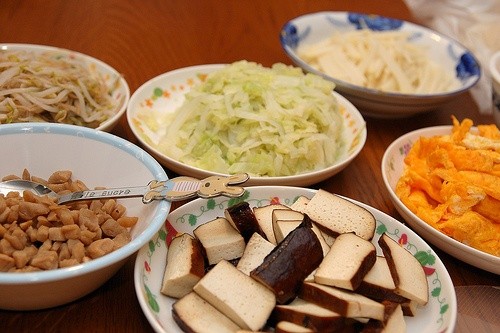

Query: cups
[489,51,500,109]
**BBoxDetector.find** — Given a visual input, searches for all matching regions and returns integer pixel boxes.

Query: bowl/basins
[0,122,172,309]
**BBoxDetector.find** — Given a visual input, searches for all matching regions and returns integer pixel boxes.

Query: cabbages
[153,61,343,176]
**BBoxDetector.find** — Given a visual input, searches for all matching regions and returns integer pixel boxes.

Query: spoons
[0,172,250,205]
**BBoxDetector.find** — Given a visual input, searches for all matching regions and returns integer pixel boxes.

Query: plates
[280,11,481,118]
[134,186,457,333]
[382,125,500,275]
[0,43,130,132]
[126,64,368,186]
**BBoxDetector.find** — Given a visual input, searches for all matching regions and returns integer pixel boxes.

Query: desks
[0,0,500,333]
